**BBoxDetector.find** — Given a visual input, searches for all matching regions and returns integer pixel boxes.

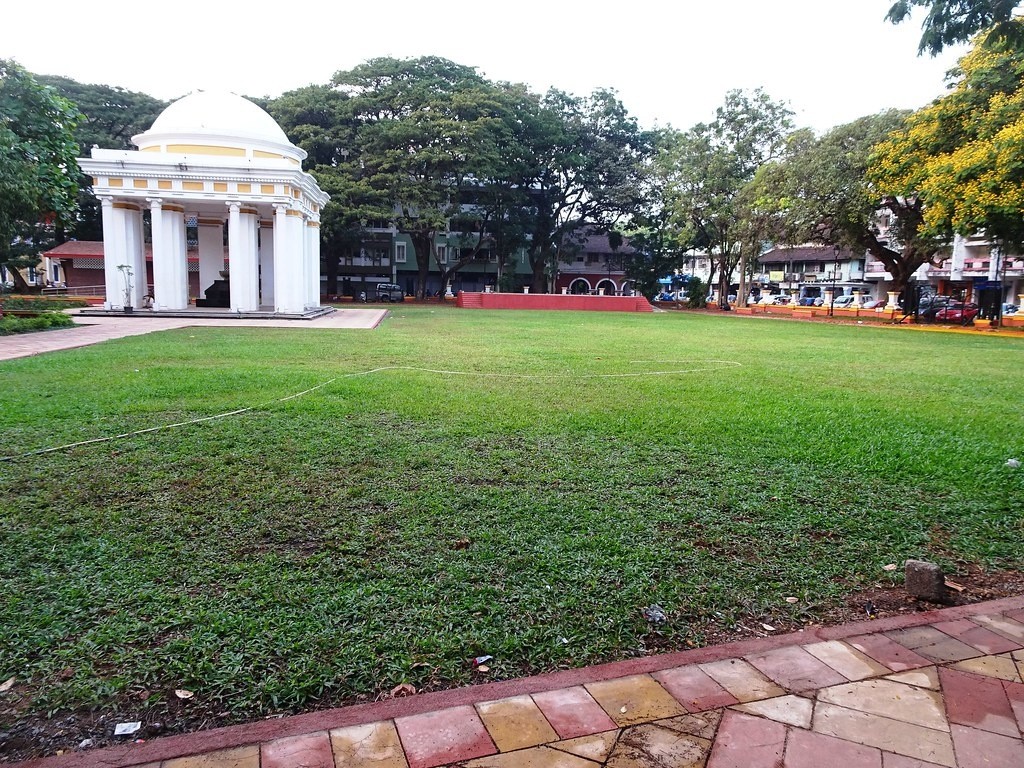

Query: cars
[861,300,886,311]
[352,292,366,302]
[653,291,692,301]
[918,296,1019,325]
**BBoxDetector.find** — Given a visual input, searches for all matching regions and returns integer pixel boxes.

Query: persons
[750,283,763,304]
[360,289,367,303]
[46,279,49,285]
[722,300,735,311]
[424,289,440,297]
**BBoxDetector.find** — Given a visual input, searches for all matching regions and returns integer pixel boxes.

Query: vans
[366,283,403,304]
[829,295,873,308]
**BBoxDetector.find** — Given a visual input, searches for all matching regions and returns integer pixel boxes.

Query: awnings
[641,274,689,283]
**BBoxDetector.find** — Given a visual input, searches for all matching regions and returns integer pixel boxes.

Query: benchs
[736,308,756,314]
[792,310,816,318]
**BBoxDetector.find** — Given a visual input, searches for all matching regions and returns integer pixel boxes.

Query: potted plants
[116,264,135,314]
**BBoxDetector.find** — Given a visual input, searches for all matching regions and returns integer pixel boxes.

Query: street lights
[28,224,40,286]
[607,256,612,295]
[549,241,557,294]
[829,245,840,316]
[989,234,1003,325]
